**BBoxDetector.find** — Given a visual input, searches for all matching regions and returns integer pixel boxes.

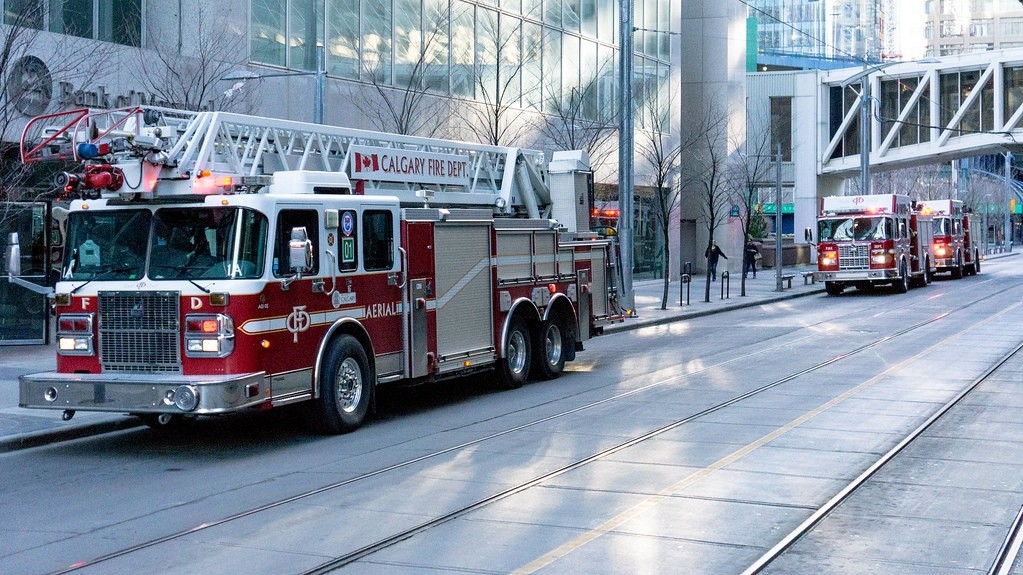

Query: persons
[745,238,759,279]
[705,240,728,281]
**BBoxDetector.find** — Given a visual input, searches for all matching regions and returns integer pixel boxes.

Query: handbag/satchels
[754,252,763,260]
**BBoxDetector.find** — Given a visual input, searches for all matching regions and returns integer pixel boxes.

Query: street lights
[220,65,325,125]
[859,57,943,196]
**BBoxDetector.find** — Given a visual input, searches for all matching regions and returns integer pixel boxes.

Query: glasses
[748,241,751,242]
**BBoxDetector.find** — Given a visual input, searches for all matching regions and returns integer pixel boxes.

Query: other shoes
[745,273,747,278]
[753,275,756,279]
[712,278,716,281]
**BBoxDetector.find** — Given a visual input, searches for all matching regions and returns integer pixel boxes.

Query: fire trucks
[803,194,934,295]
[915,199,983,278]
[16,105,639,434]
